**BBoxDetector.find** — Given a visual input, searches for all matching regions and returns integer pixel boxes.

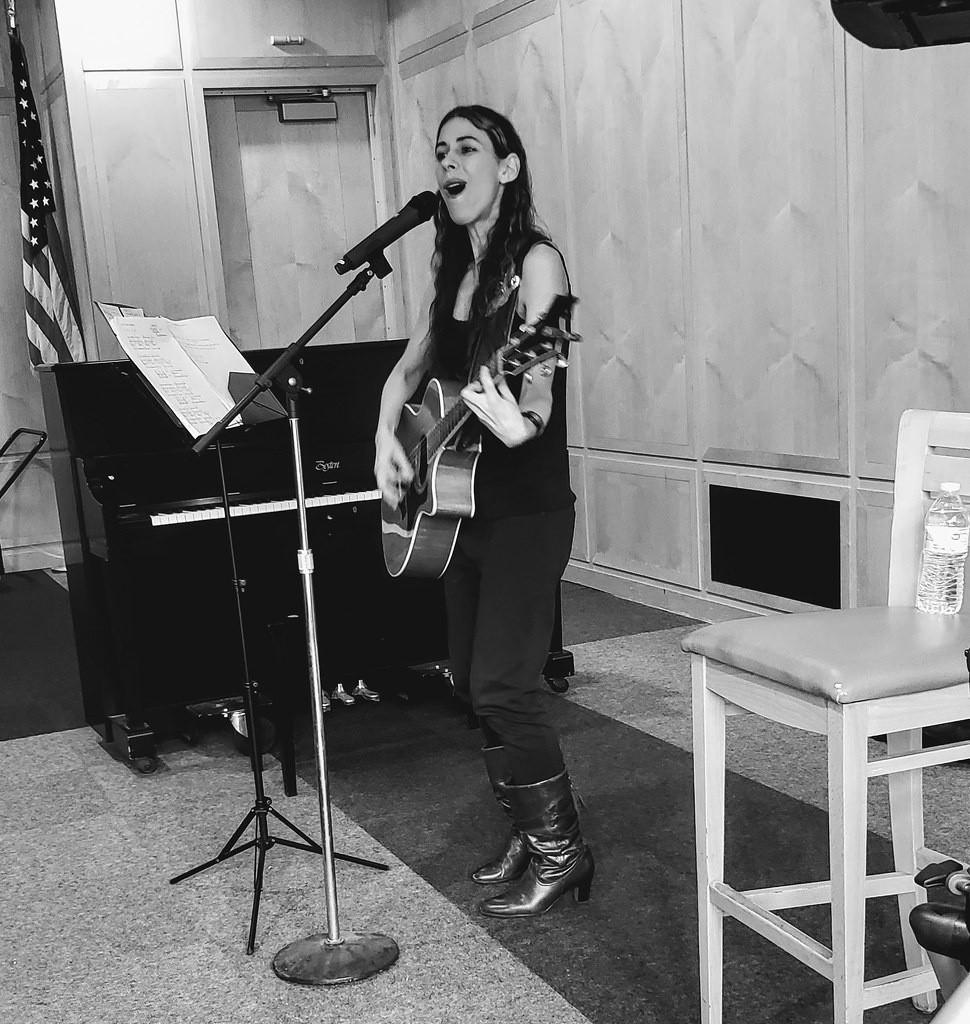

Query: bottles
[914,484,969,616]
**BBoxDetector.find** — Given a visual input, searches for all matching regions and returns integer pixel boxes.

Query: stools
[681,604,970,1024]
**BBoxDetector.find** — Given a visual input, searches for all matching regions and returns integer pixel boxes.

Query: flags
[8,29,87,383]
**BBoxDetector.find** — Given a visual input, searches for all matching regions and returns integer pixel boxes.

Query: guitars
[380,293,588,582]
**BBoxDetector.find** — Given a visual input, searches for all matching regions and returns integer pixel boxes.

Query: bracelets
[520,411,545,438]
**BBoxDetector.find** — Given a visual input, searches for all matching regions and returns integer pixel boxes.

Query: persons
[374,107,594,918]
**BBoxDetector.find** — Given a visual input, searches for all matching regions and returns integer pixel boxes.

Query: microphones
[333,191,438,275]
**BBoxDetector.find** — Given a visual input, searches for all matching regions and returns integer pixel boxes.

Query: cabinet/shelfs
[385,0,970,625]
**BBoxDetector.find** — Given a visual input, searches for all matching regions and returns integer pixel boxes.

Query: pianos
[37,324,579,770]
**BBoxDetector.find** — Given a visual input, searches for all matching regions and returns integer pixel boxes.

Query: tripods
[122,345,390,955]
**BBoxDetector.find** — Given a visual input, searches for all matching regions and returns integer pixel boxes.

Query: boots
[472,744,532,884]
[479,764,595,918]
